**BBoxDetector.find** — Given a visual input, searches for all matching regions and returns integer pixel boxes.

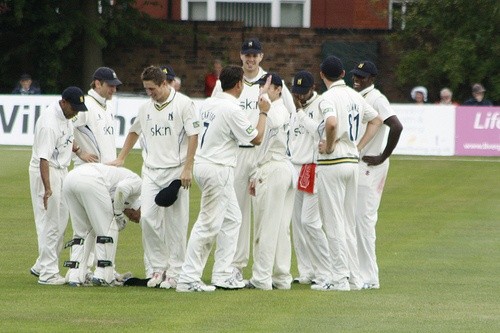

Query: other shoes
[213,271,287,290]
[146,271,163,288]
[159,277,177,289]
[36,274,68,286]
[293,276,378,291]
[176,280,217,293]
[30,267,40,279]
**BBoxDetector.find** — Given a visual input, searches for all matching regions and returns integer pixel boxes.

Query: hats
[159,63,175,81]
[93,67,122,86]
[20,74,32,82]
[62,86,89,112]
[241,39,261,55]
[471,83,487,93]
[155,178,182,208]
[349,59,378,78]
[320,56,345,77]
[255,72,282,86]
[410,86,428,102]
[290,70,315,95]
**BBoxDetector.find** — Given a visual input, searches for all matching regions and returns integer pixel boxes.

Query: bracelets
[73,145,80,152]
[258,111,268,116]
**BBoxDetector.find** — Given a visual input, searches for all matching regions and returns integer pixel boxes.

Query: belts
[240,145,255,150]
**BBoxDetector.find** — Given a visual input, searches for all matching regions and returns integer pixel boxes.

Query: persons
[410,86,427,104]
[461,83,493,105]
[159,65,183,93]
[174,65,271,292]
[433,87,459,105]
[102,65,199,289]
[204,60,222,97]
[29,86,87,287]
[212,38,296,288]
[68,66,118,286]
[63,161,143,287]
[288,71,330,291]
[306,55,381,290]
[248,72,297,291]
[347,59,402,291]
[13,74,41,94]
